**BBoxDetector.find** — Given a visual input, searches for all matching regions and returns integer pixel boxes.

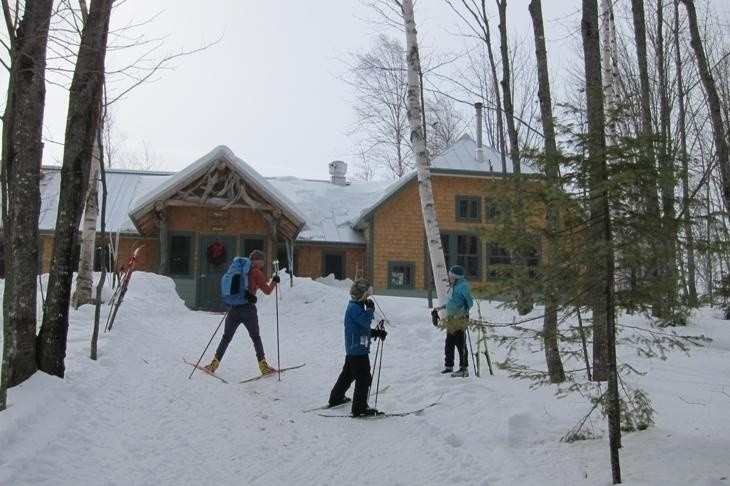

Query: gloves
[430,309,441,328]
[371,329,388,341]
[364,298,376,311]
[273,275,280,283]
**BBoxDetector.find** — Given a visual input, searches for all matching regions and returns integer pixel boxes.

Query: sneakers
[451,368,469,377]
[329,396,351,407]
[351,406,380,416]
[440,367,453,375]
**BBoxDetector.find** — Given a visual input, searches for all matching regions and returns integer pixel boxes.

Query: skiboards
[104,228,146,334]
[183,357,306,384]
[476,299,493,377]
[303,384,444,417]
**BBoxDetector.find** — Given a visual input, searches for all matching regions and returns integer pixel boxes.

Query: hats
[250,250,267,261]
[449,265,466,280]
[350,279,374,303]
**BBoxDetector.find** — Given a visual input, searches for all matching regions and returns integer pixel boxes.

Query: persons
[326,279,388,417]
[434,265,473,378]
[203,249,280,376]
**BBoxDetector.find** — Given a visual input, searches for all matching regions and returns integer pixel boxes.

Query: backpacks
[221,257,251,306]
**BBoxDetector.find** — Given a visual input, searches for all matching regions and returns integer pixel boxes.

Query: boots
[205,358,219,373]
[258,359,276,375]
